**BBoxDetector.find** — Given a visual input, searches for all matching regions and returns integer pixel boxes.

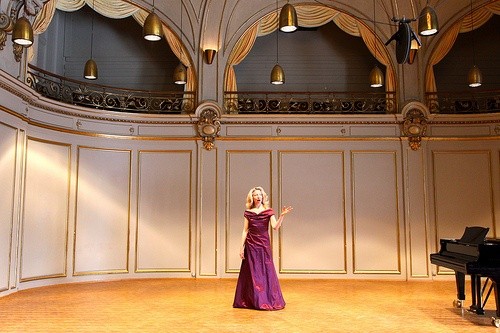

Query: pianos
[429,226,500,328]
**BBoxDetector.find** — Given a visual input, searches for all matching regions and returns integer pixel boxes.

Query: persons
[240,186,294,308]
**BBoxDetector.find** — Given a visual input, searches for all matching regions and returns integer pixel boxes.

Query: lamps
[467,0,482,88]
[173,0,188,84]
[278,0,298,32]
[12,0,35,46]
[204,49,216,65]
[271,0,286,85]
[406,49,418,64]
[142,0,163,41]
[367,0,384,88]
[417,0,439,36]
[83,0,98,80]
